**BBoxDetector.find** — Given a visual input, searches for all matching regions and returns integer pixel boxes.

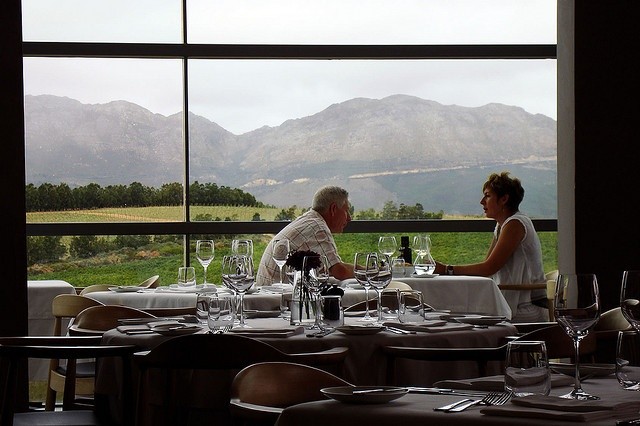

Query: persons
[255,184,366,285]
[426,172,552,323]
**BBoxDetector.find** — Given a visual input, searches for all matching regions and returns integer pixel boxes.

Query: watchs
[444,263,455,276]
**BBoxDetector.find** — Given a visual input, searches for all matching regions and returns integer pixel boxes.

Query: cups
[399,290,425,324]
[377,289,400,319]
[318,296,343,331]
[404,264,415,276]
[615,331,640,390]
[390,258,406,277]
[178,267,196,286]
[195,292,221,324]
[505,341,551,397]
[208,298,234,332]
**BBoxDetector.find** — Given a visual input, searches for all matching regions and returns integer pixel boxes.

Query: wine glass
[196,240,215,287]
[232,239,253,255]
[271,239,292,317]
[412,236,432,255]
[620,271,639,333]
[553,274,601,401]
[302,256,329,330]
[365,253,392,324]
[223,256,246,328]
[378,236,398,256]
[228,256,254,329]
[354,252,377,323]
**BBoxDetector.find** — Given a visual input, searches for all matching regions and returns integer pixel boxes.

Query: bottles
[414,233,437,274]
[398,236,412,265]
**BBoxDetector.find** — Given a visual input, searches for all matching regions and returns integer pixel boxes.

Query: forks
[446,391,511,413]
[432,392,498,412]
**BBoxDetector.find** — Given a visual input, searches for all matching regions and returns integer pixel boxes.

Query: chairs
[344,295,426,316]
[590,307,629,351]
[133,335,349,424]
[496,270,559,321]
[384,324,561,383]
[46,294,103,408]
[65,305,157,410]
[227,362,355,415]
[79,283,122,292]
[1,334,141,425]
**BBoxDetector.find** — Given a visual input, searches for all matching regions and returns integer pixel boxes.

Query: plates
[336,325,382,334]
[320,386,409,404]
[422,320,448,327]
[243,307,290,317]
[455,318,506,328]
[150,326,203,335]
[108,286,145,292]
[548,359,628,378]
[148,320,179,327]
[423,312,451,319]
[411,274,440,277]
